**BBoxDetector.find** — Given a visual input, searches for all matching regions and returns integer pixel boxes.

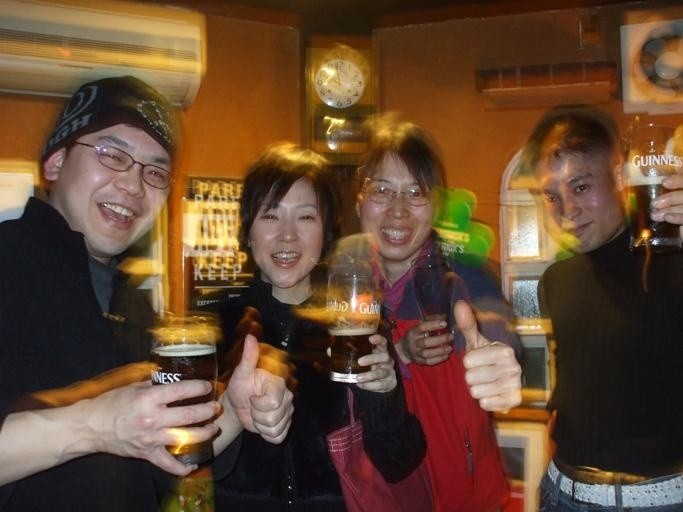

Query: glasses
[74,139,174,190]
[359,184,435,206]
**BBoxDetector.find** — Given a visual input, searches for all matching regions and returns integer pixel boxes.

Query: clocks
[298,36,380,166]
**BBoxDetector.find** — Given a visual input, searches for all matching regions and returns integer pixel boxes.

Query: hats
[37,74,174,160]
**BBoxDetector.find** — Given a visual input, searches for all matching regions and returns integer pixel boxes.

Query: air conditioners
[0,2,212,111]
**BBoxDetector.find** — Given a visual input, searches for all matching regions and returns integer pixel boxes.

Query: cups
[320,257,387,387]
[151,311,221,472]
[625,122,683,253]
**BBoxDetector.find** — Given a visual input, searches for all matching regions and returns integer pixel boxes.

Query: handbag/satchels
[325,387,432,512]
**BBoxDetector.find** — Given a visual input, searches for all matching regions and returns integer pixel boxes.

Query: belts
[546,460,682,508]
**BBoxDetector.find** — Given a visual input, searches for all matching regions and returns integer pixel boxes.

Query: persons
[0,76,294,512]
[332,121,524,511]
[521,110,682,511]
[184,141,427,511]
